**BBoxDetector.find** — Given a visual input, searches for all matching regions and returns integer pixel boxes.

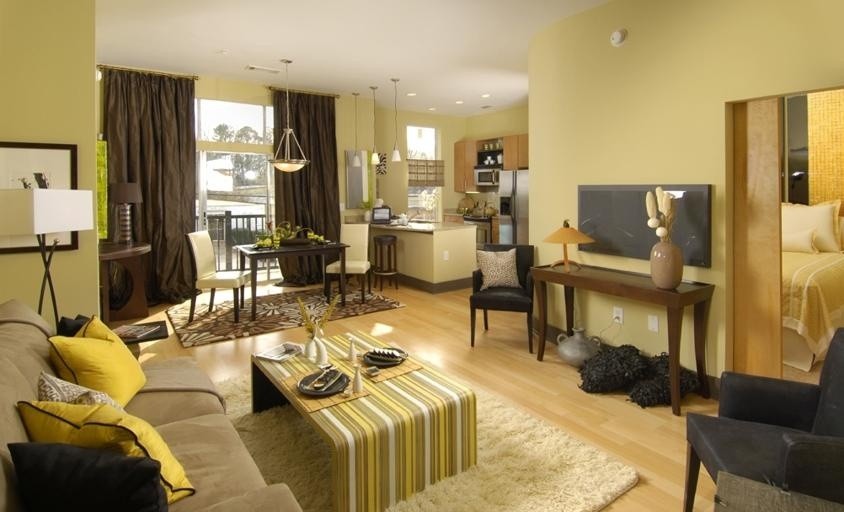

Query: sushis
[313,368,339,390]
[368,347,402,361]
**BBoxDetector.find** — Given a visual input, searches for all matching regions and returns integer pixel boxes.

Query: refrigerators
[499,170,529,244]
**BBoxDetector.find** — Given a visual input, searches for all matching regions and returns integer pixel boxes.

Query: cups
[484,143,503,165]
[464,208,470,216]
[273,233,281,250]
[402,219,408,226]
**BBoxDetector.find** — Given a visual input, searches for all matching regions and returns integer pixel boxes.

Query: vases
[650,241,682,290]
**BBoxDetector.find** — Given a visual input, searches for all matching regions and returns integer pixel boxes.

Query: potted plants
[282,293,366,395]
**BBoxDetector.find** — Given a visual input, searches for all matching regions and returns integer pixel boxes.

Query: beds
[781,248,844,372]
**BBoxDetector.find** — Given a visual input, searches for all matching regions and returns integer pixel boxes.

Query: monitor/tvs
[576,184,712,268]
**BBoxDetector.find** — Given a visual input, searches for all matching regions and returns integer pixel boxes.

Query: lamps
[268,58,310,173]
[350,80,401,167]
[108,179,144,250]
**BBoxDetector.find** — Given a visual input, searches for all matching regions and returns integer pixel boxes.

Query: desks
[99,244,154,324]
[530,262,715,415]
[237,227,350,317]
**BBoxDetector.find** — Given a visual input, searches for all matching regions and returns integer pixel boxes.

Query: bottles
[352,363,363,393]
[348,337,358,361]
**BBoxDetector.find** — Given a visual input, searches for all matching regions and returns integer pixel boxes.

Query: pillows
[7,314,196,512]
[476,248,517,288]
[780,197,844,252]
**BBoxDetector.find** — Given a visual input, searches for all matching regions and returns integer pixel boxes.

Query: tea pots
[472,202,498,217]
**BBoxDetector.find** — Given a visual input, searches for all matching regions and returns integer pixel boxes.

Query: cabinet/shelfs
[453,131,529,191]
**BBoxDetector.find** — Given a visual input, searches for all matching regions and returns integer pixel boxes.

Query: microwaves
[473,169,501,186]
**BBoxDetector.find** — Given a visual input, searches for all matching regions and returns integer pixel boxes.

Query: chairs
[684,327,843,510]
[470,246,534,353]
[186,230,250,322]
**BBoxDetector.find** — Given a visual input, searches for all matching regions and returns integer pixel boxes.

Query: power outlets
[612,306,622,322]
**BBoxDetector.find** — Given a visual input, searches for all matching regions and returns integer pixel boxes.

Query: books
[255,342,305,361]
[112,325,161,339]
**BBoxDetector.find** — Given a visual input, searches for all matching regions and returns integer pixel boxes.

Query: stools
[325,222,372,302]
[372,234,400,290]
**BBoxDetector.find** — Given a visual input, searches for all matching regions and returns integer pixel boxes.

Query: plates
[254,246,272,250]
[364,347,407,366]
[299,371,350,397]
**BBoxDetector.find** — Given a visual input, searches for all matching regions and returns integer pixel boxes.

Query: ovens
[464,221,491,250]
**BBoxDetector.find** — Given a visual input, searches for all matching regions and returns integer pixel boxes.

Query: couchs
[1,293,303,512]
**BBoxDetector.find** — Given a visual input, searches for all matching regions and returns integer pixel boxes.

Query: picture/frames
[0,140,79,256]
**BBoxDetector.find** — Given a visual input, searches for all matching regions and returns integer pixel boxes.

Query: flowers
[646,187,676,237]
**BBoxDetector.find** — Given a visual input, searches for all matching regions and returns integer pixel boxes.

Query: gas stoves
[463,215,492,221]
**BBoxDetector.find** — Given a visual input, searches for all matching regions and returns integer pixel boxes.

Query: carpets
[213,356,638,512]
[164,282,403,348]
